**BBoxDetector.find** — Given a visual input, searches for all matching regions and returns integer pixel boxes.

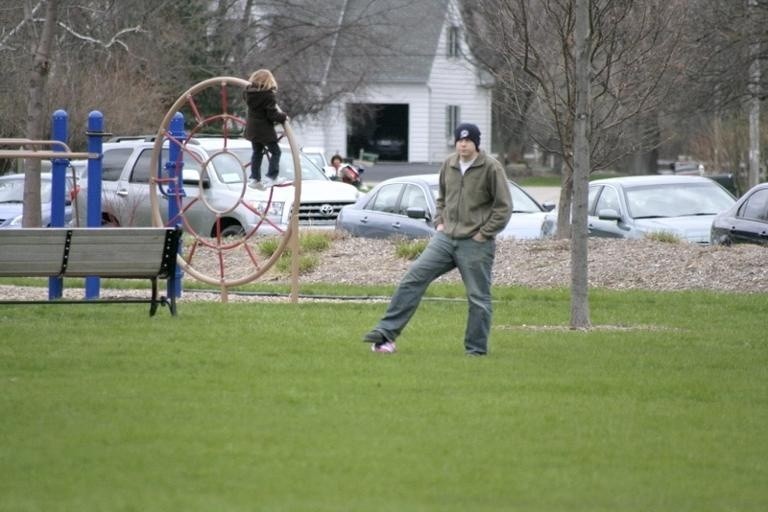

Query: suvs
[68,129,360,240]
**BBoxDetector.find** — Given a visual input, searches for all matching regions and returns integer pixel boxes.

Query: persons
[330,154,360,187]
[241,67,288,191]
[362,122,514,357]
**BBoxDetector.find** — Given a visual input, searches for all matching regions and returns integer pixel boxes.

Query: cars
[369,133,405,157]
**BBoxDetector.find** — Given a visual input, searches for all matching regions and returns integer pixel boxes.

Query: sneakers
[246,178,267,192]
[260,175,288,189]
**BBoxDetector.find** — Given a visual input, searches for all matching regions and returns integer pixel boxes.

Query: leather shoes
[361,329,386,345]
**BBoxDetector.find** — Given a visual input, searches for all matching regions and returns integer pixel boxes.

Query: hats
[453,122,483,148]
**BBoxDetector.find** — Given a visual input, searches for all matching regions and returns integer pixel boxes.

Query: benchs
[0,222,185,320]
